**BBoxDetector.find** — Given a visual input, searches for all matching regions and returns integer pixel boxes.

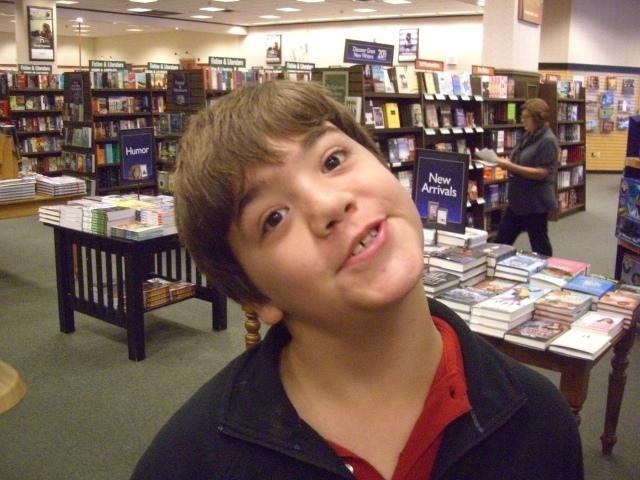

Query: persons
[267,41,281,58]
[128,77,584,478]
[39,22,52,41]
[403,33,414,54]
[494,100,561,256]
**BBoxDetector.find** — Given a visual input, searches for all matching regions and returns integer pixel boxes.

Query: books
[366,65,521,231]
[0,177,36,203]
[93,277,195,310]
[39,194,178,242]
[422,227,639,363]
[538,76,635,209]
[0,64,310,194]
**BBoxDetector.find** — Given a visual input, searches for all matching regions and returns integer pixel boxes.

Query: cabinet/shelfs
[0,64,587,245]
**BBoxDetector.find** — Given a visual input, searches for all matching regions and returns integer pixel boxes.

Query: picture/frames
[26,5,55,62]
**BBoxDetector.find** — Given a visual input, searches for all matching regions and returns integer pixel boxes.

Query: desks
[43,222,227,361]
[472,324,637,455]
